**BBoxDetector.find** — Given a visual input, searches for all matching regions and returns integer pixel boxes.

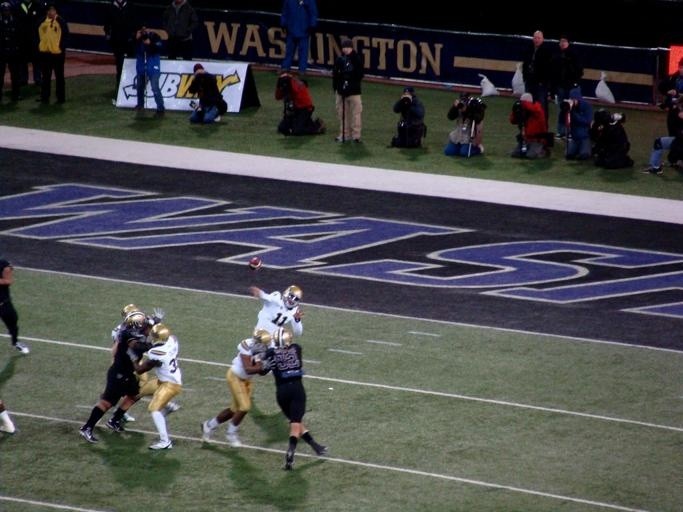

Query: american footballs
[250,256,262,269]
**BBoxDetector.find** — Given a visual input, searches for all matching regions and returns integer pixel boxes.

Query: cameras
[458,99,468,112]
[189,100,203,114]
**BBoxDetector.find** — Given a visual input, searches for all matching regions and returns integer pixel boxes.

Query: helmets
[122,304,169,346]
[257,327,293,348]
[284,285,302,309]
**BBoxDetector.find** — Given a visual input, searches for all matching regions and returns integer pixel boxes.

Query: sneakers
[201,421,215,446]
[641,166,663,175]
[14,342,30,354]
[79,427,98,444]
[148,440,172,449]
[106,412,135,431]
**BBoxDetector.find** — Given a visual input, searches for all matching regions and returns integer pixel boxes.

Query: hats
[571,87,581,97]
[521,93,533,103]
[341,39,353,47]
[194,64,203,71]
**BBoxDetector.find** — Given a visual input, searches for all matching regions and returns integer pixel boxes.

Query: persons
[0,258,30,354]
[247,285,305,375]
[0,400,15,433]
[251,327,328,470]
[78,304,182,449]
[200,329,276,448]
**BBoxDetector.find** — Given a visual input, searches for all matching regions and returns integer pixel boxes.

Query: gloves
[262,357,276,371]
